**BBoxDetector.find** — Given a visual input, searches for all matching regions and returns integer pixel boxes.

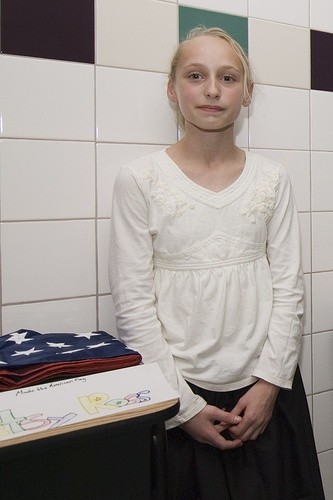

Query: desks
[0,361,181,500]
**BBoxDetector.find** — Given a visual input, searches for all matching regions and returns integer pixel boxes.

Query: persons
[108,26,324,500]
